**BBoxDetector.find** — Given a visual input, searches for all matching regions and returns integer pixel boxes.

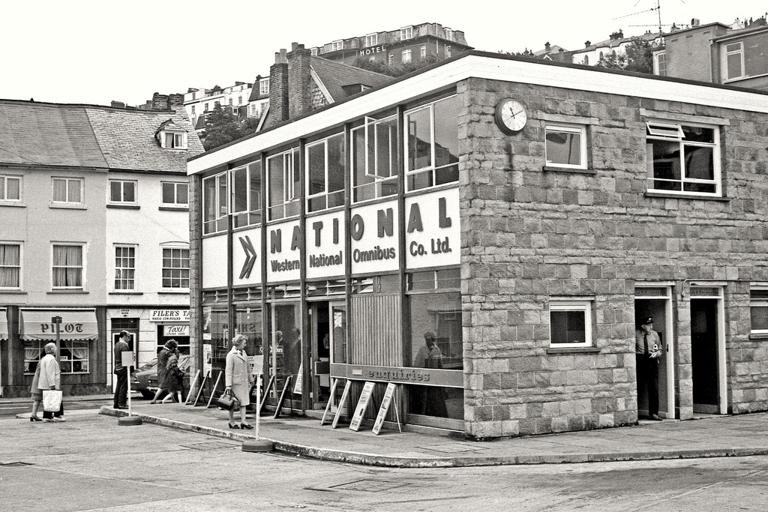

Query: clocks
[494,97,528,136]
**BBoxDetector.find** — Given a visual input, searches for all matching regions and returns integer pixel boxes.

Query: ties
[644,334,648,354]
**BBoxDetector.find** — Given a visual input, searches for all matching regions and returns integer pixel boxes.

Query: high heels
[229,423,239,429]
[241,424,253,429]
[30,415,42,421]
[53,415,66,423]
[42,418,54,423]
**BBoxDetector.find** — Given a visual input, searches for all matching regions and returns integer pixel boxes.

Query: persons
[290,328,301,373]
[151,339,177,403]
[159,355,183,404]
[277,331,288,357]
[38,342,66,422]
[413,332,448,418]
[113,330,129,409]
[637,316,663,420]
[225,334,254,429]
[30,353,47,422]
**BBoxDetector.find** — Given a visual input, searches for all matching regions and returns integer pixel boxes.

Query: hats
[646,315,653,325]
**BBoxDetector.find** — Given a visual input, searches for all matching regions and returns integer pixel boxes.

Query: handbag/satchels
[43,390,62,412]
[217,390,240,410]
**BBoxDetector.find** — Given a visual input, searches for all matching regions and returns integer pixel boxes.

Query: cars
[147,355,191,401]
[131,358,158,398]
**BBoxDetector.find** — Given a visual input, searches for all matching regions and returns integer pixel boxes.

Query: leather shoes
[653,414,662,421]
